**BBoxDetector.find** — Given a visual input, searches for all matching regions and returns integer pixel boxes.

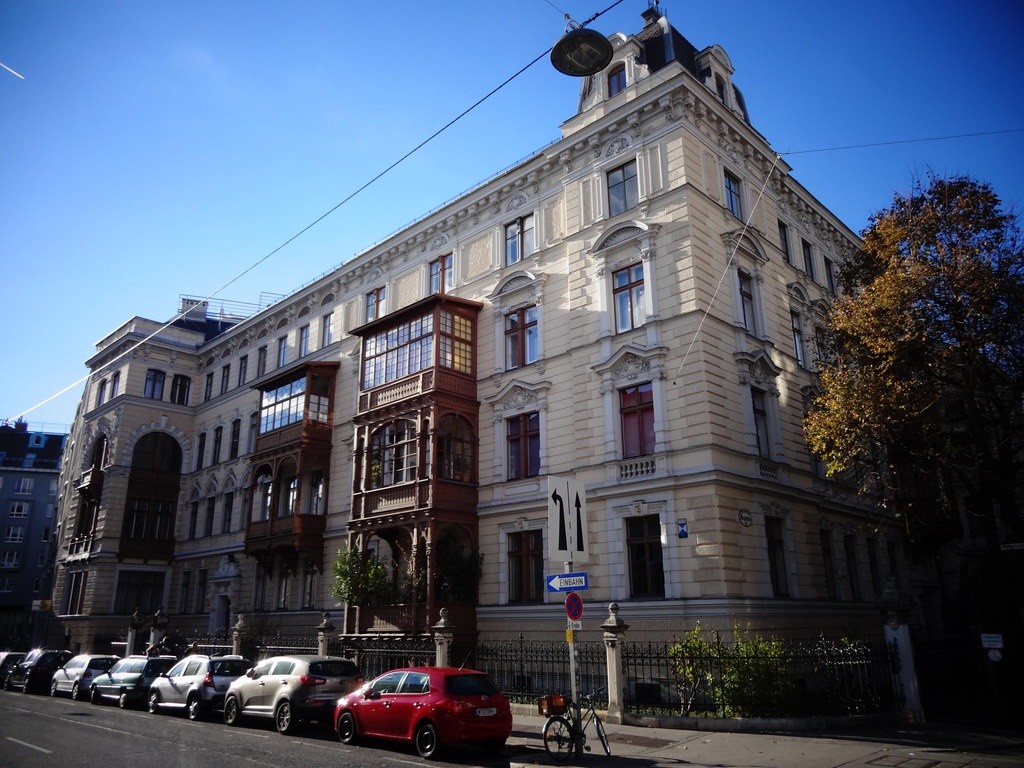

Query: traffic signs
[546,573,589,592]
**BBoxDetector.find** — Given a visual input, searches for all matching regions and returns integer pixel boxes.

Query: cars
[3,649,77,695]
[0,650,27,689]
[50,654,122,700]
[88,653,181,709]
[223,654,366,735]
[146,652,255,721]
[332,667,514,758]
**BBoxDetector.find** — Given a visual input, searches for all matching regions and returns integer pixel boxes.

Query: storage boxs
[537,696,566,715]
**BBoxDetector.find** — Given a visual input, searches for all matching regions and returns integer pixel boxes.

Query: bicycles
[541,686,613,760]
